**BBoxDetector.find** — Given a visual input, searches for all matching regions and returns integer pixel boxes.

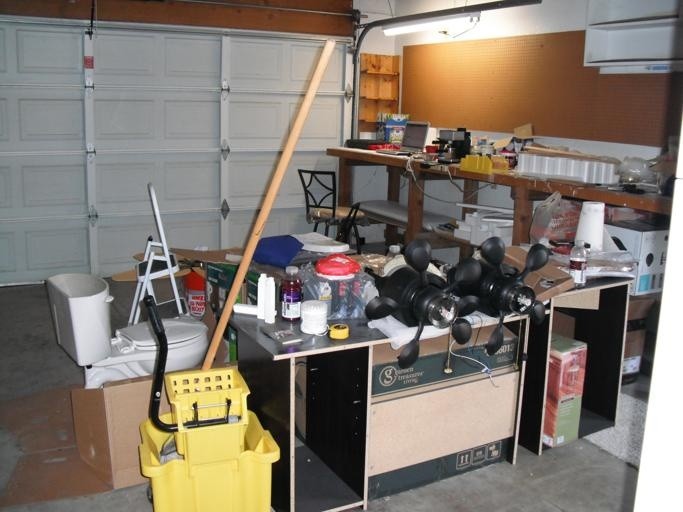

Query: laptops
[376,121,430,155]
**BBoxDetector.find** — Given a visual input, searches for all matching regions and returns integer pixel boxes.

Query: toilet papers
[299,300,329,336]
[576,202,606,252]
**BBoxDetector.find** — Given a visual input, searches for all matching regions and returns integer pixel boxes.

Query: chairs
[336,203,360,246]
[298,169,365,254]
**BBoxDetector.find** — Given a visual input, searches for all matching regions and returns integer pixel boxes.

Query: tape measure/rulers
[326,323,349,340]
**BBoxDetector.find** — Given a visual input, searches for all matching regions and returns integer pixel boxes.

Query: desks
[228,311,532,512]
[323,145,673,258]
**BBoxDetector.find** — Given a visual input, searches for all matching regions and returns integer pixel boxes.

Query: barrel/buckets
[184,270,206,319]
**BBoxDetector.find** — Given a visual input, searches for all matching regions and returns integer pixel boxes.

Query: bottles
[569,239,588,288]
[279,261,331,337]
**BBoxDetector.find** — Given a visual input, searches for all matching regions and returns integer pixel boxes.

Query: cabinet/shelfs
[583,17,683,75]
[359,53,399,138]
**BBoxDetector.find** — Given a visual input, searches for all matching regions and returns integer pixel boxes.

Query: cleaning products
[282,265,303,322]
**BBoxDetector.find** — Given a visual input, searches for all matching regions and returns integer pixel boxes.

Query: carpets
[582,392,647,468]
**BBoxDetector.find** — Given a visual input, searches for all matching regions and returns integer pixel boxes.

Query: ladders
[129,183,189,326]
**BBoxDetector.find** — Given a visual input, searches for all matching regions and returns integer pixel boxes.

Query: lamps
[381,11,483,37]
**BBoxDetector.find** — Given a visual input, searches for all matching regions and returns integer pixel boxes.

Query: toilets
[43,271,210,389]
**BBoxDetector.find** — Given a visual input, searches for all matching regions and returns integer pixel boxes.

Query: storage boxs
[369,324,520,500]
[622,324,646,385]
[602,219,669,296]
[69,364,241,492]
[377,111,409,145]
